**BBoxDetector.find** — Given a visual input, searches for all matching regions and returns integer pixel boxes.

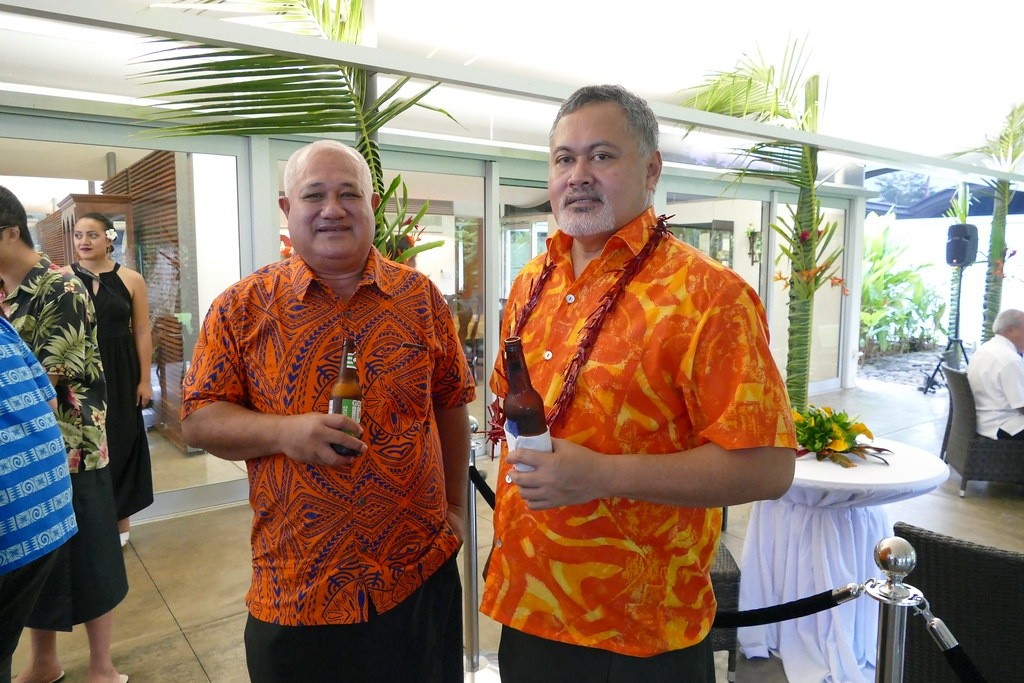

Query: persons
[965,309,1024,442]
[476,81,800,683]
[0,179,153,683]
[176,137,478,683]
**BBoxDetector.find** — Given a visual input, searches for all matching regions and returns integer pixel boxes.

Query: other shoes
[119,531,131,547]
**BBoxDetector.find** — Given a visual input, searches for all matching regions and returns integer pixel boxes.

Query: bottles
[503,338,547,439]
[328,338,361,457]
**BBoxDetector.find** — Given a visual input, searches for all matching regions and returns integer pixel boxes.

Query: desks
[450,313,484,346]
[735,436,950,683]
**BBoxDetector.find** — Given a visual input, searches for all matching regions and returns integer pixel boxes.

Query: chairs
[456,308,482,385]
[894,522,1024,683]
[711,544,740,683]
[939,362,1024,498]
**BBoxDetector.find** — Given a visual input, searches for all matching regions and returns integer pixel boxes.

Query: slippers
[47,671,65,683]
[117,673,130,683]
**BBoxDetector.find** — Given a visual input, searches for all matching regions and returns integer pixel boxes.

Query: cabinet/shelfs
[57,195,137,271]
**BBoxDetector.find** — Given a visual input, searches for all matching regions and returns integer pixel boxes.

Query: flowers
[386,216,427,266]
[106,229,118,240]
[995,247,1017,279]
[791,403,895,467]
[773,229,850,299]
